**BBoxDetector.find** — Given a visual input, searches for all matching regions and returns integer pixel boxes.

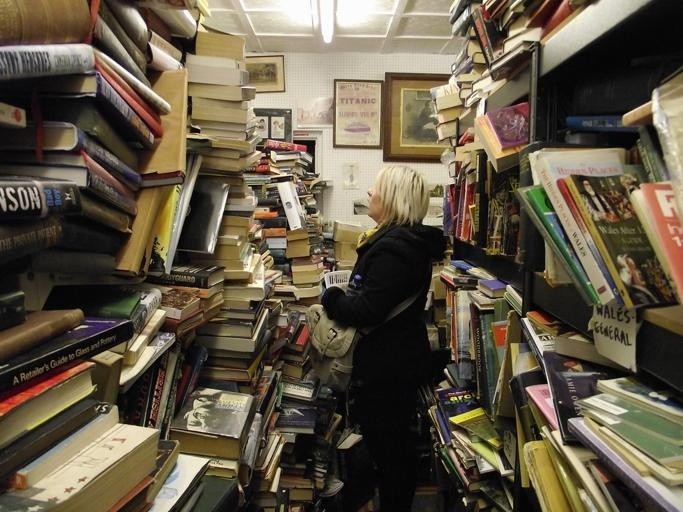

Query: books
[429,1,683,512]
[1,0,341,512]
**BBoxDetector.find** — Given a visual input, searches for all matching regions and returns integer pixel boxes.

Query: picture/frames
[332,78,386,149]
[244,55,285,94]
[385,70,452,163]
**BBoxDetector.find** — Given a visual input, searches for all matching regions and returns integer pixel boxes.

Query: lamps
[317,0,334,43]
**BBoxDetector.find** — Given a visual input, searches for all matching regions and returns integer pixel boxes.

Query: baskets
[324,270,352,294]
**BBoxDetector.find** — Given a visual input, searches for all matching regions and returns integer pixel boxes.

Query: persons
[321,165,447,512]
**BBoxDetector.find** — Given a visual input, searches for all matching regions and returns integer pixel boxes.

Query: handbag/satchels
[304,304,360,393]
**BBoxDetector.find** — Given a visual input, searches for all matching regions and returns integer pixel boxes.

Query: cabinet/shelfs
[440,1,682,512]
